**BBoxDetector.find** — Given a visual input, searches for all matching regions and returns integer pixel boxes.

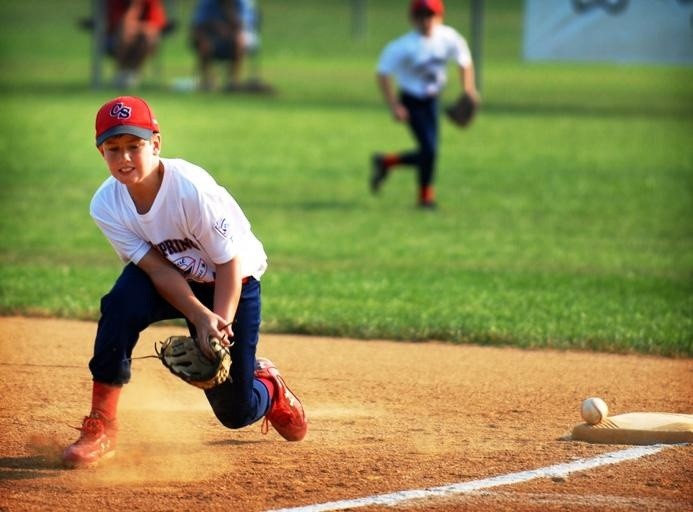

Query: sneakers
[368,153,388,196]
[256,357,307,442]
[59,411,118,470]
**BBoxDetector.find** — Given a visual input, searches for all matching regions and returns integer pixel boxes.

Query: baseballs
[580,396,607,425]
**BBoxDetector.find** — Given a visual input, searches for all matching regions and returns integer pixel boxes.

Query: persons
[91,0,180,88]
[186,0,264,96]
[63,93,306,468]
[363,1,484,212]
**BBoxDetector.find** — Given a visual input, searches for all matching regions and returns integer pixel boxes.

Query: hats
[95,95,160,146]
[409,1,443,16]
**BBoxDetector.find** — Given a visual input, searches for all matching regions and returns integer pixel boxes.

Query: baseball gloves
[154,335,234,389]
[443,96,478,128]
